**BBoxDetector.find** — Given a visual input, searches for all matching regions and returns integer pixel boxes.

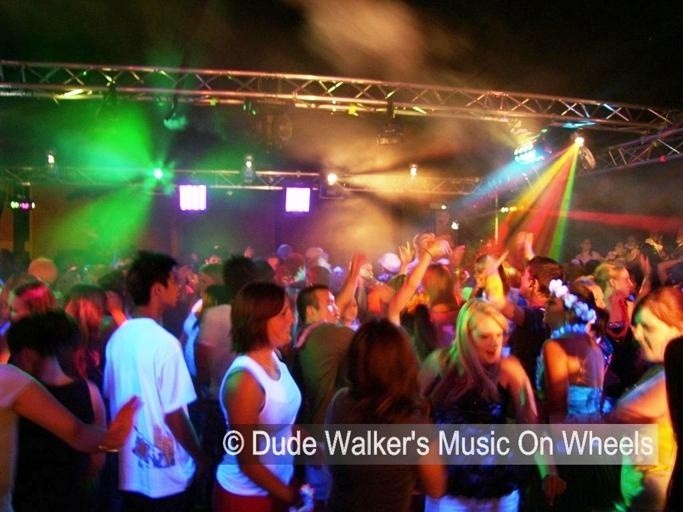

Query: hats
[305,247,330,259]
[376,254,402,273]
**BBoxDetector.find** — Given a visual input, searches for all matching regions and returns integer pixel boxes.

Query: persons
[0,229,682,511]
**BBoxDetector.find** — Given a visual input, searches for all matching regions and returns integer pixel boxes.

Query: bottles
[104,396,141,452]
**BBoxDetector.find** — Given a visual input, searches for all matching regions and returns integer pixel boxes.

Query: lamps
[568,130,586,148]
